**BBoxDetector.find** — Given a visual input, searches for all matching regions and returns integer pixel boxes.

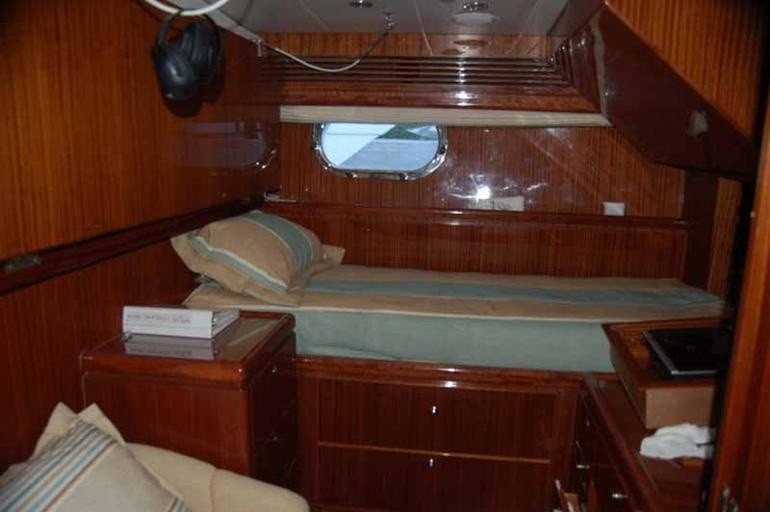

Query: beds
[179,263,725,373]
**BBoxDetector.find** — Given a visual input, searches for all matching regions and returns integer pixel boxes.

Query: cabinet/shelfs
[551,374,716,512]
[295,360,582,511]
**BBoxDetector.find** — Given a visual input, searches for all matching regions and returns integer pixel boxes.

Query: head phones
[151,8,225,119]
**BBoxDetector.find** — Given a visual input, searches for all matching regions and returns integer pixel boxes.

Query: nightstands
[78,309,298,494]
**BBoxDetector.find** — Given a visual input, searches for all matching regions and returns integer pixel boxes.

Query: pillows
[1,400,199,511]
[170,209,347,309]
